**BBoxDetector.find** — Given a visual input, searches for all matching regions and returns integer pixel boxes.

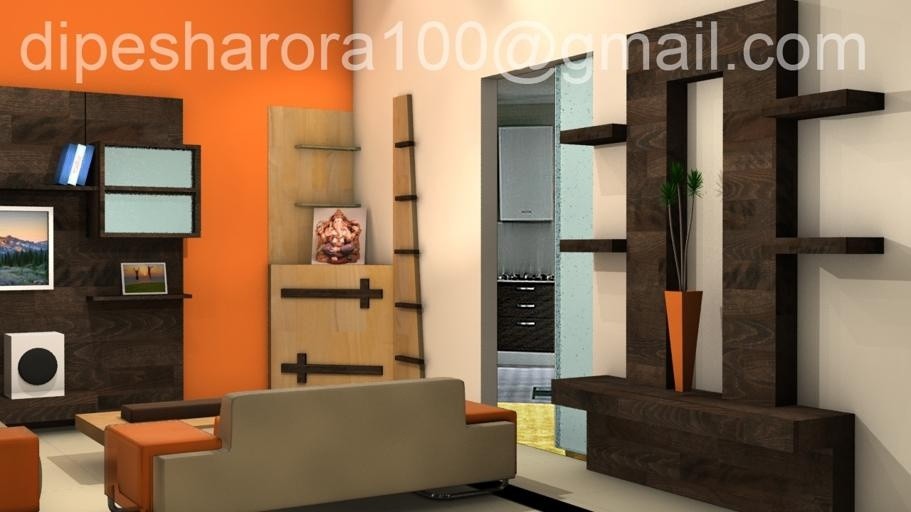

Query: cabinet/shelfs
[267,94,426,388]
[89,140,201,239]
[551,1,885,511]
[497,274,556,352]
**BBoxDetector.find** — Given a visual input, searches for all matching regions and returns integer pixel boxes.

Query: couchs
[0,421,42,512]
[103,377,517,512]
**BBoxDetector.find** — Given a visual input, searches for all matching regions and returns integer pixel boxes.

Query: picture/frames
[0,206,55,291]
[120,262,168,296]
[311,208,367,264]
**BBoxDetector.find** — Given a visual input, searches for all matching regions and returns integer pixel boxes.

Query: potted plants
[658,160,704,394]
